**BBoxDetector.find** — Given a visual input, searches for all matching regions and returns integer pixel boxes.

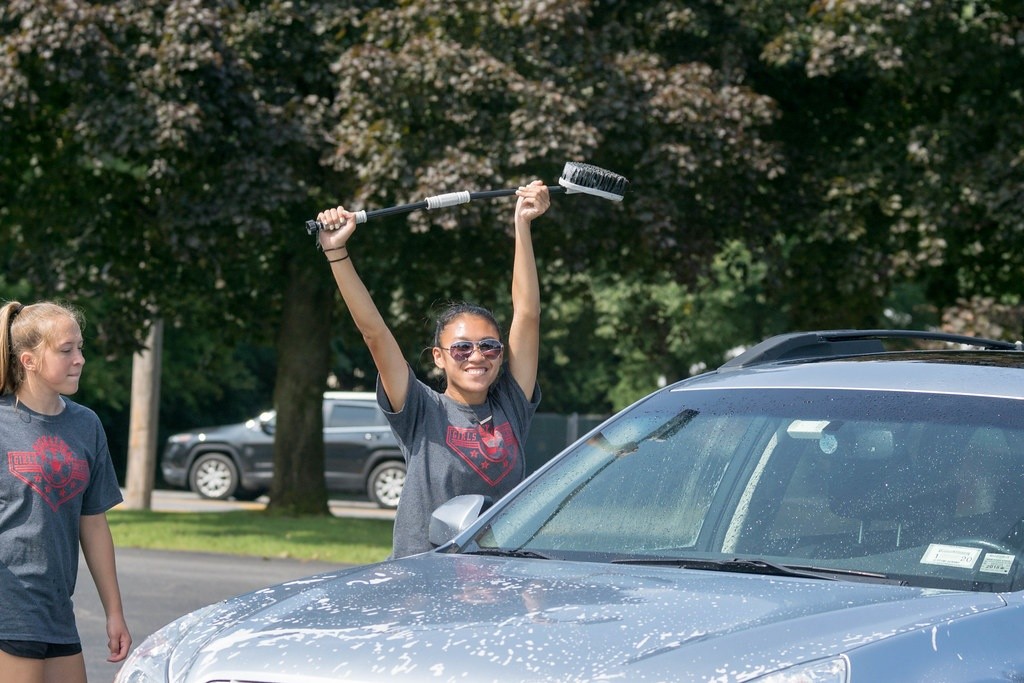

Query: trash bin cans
[526,413,612,479]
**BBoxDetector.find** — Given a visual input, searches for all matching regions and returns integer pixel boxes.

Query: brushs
[304,162,631,238]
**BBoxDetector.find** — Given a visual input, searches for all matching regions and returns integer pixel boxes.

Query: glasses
[440,339,505,361]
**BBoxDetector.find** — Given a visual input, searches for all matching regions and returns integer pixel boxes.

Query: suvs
[111,326,1023,683]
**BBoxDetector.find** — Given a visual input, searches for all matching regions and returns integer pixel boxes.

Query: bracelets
[327,254,349,263]
[322,246,346,252]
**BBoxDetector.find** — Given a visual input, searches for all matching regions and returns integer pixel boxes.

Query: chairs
[785,459,939,560]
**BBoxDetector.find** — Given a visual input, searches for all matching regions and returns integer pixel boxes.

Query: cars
[161,389,408,510]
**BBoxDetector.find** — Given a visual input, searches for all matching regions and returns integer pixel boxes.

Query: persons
[315,180,550,562]
[0,302,132,683]
